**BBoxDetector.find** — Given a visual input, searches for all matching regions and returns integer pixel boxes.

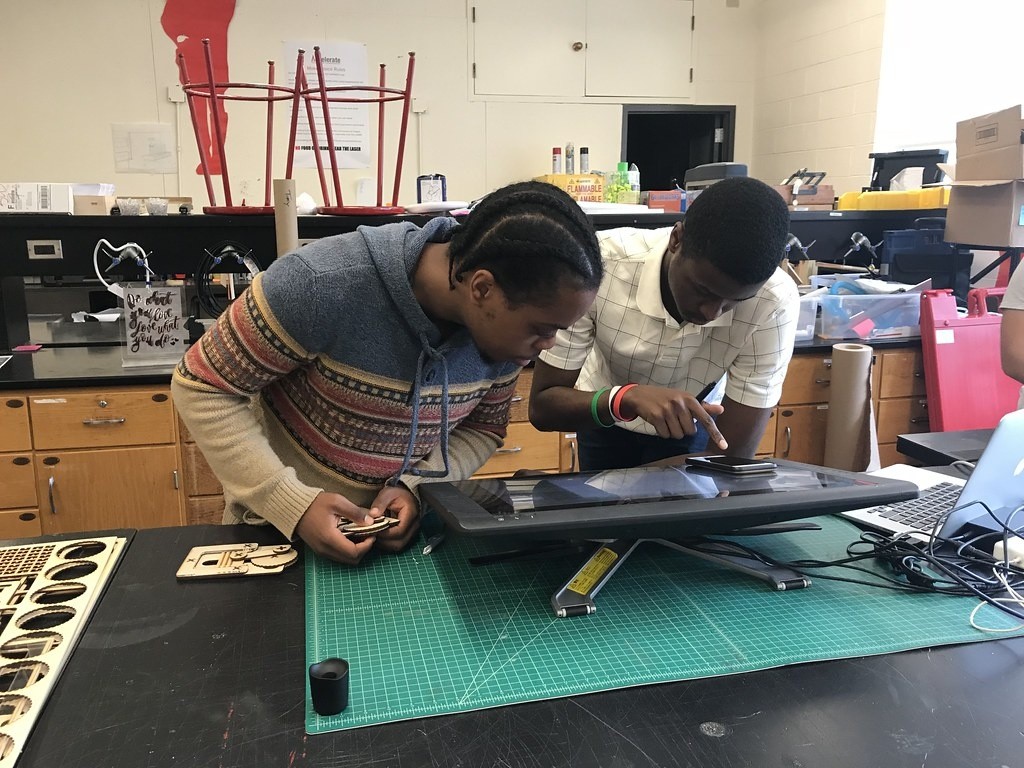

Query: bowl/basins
[143,198,171,215]
[115,198,143,215]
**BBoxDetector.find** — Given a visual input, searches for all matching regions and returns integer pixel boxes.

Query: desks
[0,431,1024,768]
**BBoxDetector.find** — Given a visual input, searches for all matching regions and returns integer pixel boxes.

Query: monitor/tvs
[416,463,921,617]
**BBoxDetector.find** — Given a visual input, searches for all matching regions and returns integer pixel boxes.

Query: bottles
[565,144,574,174]
[579,147,589,174]
[552,147,561,174]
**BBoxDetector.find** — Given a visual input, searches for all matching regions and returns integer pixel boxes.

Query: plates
[404,201,469,214]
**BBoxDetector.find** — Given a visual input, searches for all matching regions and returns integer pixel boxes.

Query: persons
[999,256,1024,410]
[170,180,603,563]
[529,176,801,471]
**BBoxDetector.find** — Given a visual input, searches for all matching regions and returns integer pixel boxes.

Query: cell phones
[684,456,776,471]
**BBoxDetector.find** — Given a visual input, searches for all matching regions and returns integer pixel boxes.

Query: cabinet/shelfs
[0,320,933,539]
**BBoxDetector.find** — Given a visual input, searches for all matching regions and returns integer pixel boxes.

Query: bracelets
[591,384,639,428]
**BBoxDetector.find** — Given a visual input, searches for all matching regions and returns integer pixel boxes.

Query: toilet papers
[272,177,299,260]
[822,342,874,471]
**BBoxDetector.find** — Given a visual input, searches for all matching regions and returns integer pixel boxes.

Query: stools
[297,46,415,215]
[178,39,305,213]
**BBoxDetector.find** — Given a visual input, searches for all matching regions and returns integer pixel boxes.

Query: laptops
[830,409,1024,549]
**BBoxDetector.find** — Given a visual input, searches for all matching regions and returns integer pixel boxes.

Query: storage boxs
[794,296,821,342]
[955,104,1024,180]
[920,163,1024,247]
[808,274,872,290]
[73,195,116,215]
[815,292,920,340]
[533,175,604,203]
